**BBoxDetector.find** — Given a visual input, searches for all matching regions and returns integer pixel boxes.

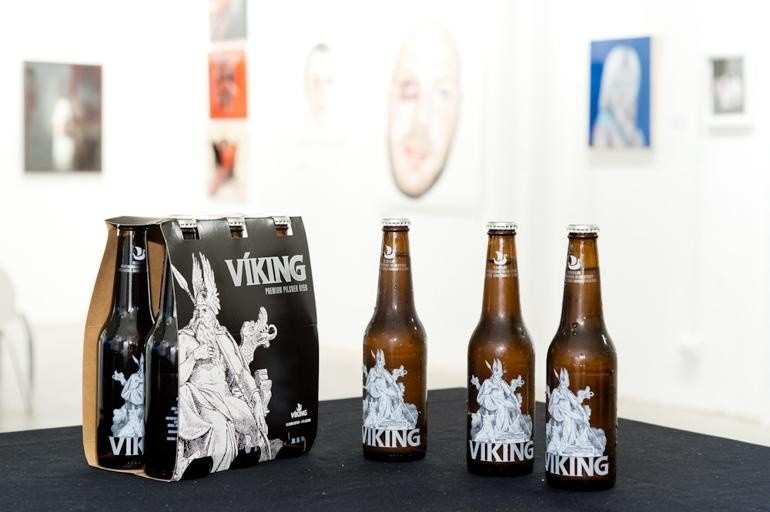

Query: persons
[381,27,463,202]
[304,41,333,128]
[583,48,644,148]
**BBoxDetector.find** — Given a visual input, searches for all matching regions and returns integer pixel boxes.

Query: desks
[1,386,770,511]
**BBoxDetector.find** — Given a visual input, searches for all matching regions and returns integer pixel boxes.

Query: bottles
[467,223,535,476]
[275,223,288,237]
[542,225,618,492]
[360,219,429,462]
[142,245,176,480]
[180,226,199,241]
[229,226,243,238]
[95,225,155,470]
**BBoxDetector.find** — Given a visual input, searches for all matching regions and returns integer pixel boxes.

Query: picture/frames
[583,29,658,160]
[702,47,756,125]
